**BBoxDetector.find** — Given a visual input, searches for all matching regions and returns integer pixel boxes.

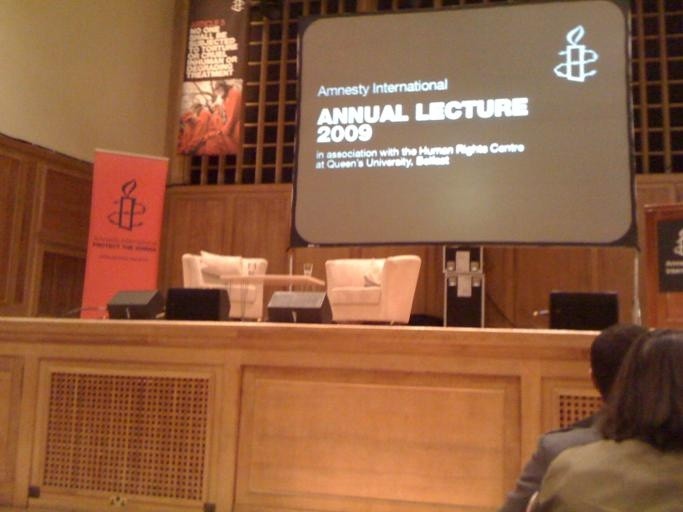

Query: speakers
[165,288,231,320]
[267,291,332,323]
[107,289,164,319]
[548,292,620,330]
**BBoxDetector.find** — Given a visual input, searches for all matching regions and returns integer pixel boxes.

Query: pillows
[200,250,244,277]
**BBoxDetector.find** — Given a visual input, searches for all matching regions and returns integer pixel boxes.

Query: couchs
[325,254,422,325]
[182,254,268,322]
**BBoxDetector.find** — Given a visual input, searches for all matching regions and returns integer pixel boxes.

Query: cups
[247,262,256,276]
[302,262,313,277]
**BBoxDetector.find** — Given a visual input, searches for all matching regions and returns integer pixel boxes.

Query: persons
[177,78,242,156]
[498,321,650,512]
[526,325,683,511]
